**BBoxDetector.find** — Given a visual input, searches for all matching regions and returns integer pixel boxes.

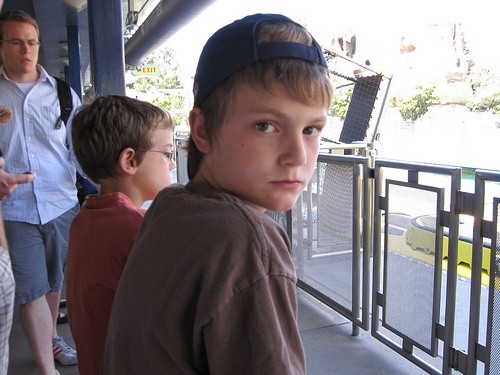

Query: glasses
[134,148,177,160]
[0,39,41,47]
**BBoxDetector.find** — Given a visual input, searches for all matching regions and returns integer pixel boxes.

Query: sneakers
[53,336,78,365]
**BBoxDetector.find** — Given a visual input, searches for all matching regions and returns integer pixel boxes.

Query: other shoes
[57,312,68,324]
[59,298,66,308]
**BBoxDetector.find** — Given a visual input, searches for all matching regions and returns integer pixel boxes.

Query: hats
[186,13,329,180]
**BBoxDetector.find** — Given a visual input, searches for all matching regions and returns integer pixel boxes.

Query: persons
[0,155,36,375]
[66,95,176,375]
[0,8,82,375]
[102,13,334,375]
